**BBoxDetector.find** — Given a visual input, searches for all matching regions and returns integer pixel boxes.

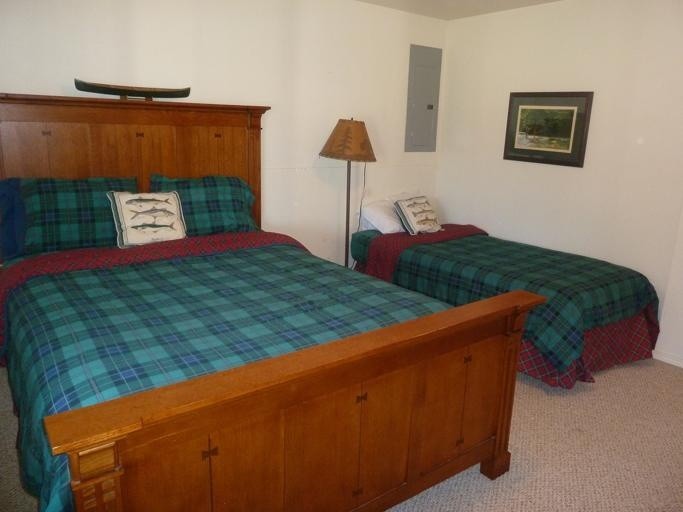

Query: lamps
[317,113,377,275]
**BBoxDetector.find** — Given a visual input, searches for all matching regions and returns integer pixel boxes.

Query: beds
[351,225,663,388]
[1,92,547,510]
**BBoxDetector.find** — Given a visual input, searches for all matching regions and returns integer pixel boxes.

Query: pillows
[357,200,404,235]
[149,172,261,235]
[105,188,188,251]
[392,194,444,235]
[14,175,143,250]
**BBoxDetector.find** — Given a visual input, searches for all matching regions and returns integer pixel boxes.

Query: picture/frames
[502,87,594,170]
